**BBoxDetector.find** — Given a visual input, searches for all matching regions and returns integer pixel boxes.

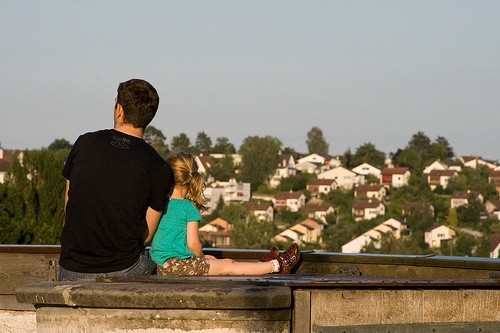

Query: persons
[57,79,174,281]
[149,151,301,276]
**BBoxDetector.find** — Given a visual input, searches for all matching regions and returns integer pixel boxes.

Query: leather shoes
[260,247,279,262]
[276,243,300,274]
